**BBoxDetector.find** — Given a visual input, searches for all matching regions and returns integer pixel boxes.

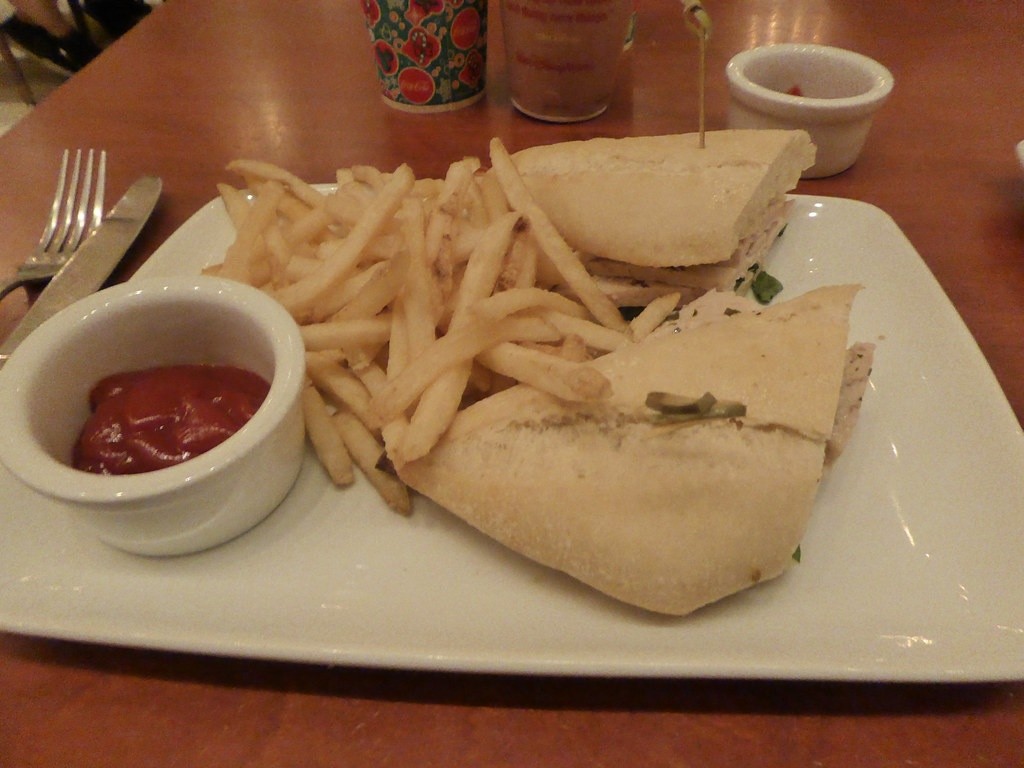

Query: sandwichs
[374,282,870,620]
[480,129,816,308]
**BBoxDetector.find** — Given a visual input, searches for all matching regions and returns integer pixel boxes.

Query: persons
[0,0,153,77]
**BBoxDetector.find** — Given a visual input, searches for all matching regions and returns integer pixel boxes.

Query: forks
[0,147,110,306]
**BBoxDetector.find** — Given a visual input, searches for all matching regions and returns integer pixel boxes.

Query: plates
[0,183,1024,685]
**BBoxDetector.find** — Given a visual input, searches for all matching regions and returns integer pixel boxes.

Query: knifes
[0,172,164,372]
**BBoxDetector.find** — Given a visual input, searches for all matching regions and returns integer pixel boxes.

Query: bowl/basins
[725,42,895,180]
[0,273,307,559]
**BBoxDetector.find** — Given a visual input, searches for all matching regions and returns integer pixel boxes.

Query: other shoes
[83,0,155,41]
[0,14,103,76]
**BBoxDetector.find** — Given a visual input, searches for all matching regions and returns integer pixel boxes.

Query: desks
[1,0,1024,768]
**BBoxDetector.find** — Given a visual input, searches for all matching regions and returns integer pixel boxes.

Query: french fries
[187,135,683,520]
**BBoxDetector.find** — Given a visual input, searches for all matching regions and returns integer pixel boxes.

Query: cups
[498,1,634,124]
[357,0,490,116]
